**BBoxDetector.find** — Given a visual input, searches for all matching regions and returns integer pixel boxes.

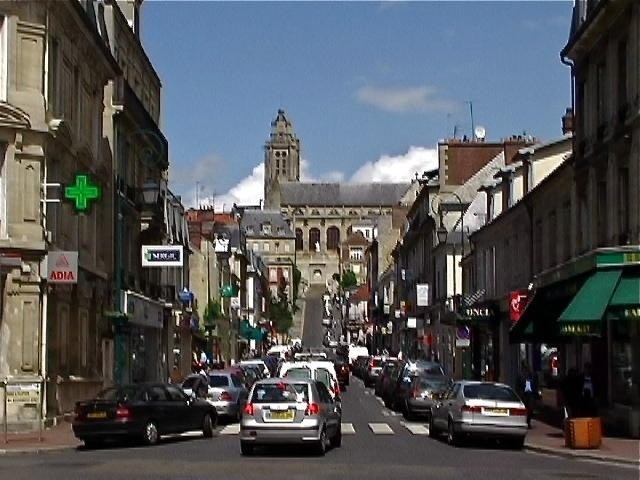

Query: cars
[71,382,216,446]
[225,338,454,420]
[181,371,250,425]
[321,294,332,326]
[429,380,529,451]
[239,378,344,457]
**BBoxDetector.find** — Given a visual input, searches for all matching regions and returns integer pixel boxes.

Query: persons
[521,370,544,430]
[555,361,609,430]
[191,364,212,399]
[376,344,398,358]
[285,340,303,362]
[240,345,261,360]
[192,343,207,373]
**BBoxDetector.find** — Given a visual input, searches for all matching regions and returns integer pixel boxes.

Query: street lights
[431,191,467,380]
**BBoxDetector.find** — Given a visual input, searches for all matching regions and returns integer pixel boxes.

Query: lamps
[135,177,159,211]
[560,105,576,134]
